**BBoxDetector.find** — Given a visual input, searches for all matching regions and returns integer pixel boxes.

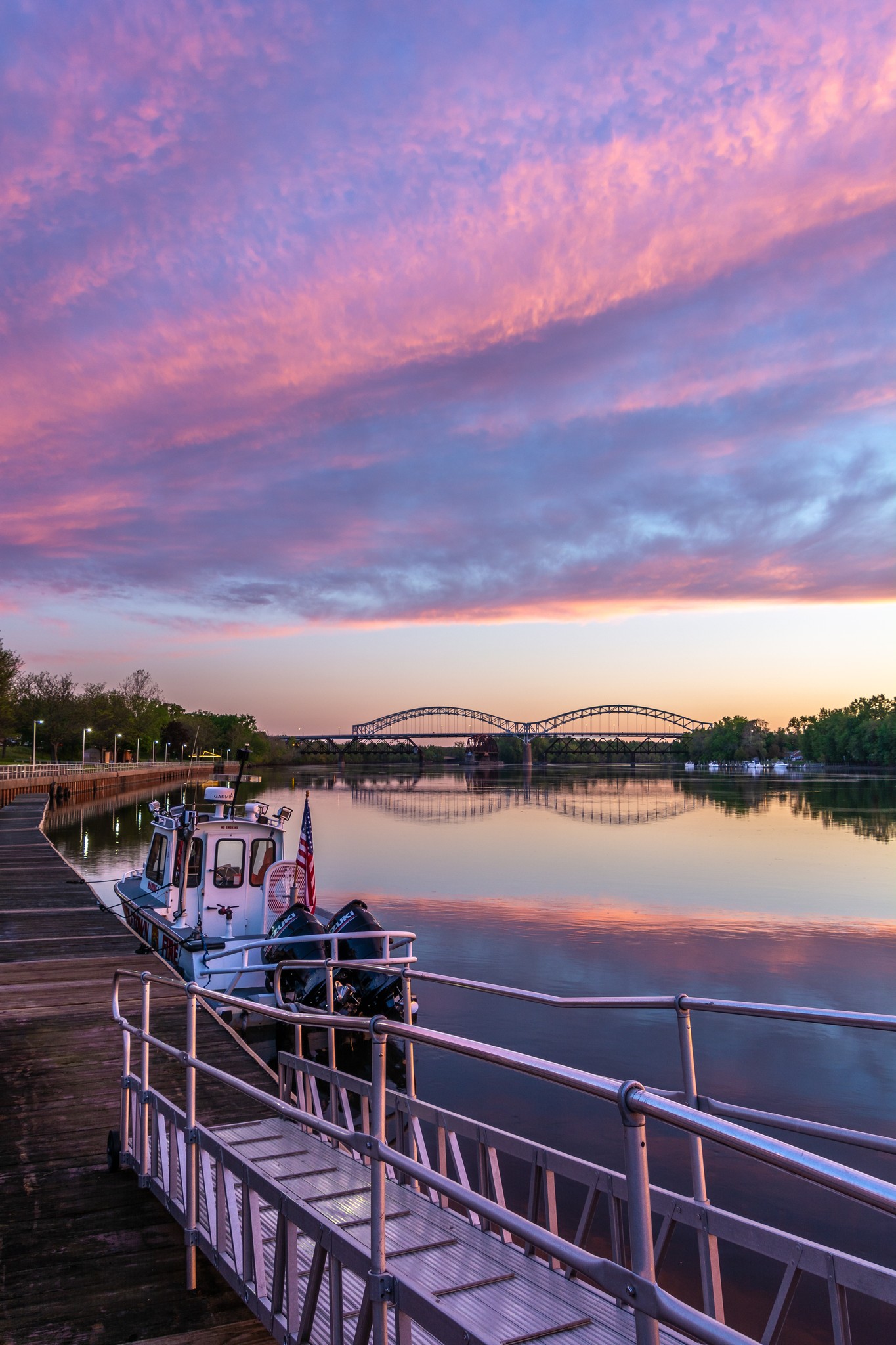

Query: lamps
[182,810,199,826]
[244,801,269,818]
[168,804,186,818]
[147,800,160,813]
[272,807,293,828]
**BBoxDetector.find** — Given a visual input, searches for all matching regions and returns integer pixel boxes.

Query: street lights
[220,749,224,764]
[613,724,615,733]
[669,725,670,733]
[114,734,122,767]
[441,725,443,733]
[182,744,187,763]
[82,728,92,768]
[560,724,561,733]
[469,725,471,733]
[153,740,159,765]
[165,743,171,764]
[493,724,495,733]
[585,724,587,733]
[299,728,300,736]
[338,727,340,735]
[32,720,44,770]
[227,749,231,762]
[136,738,143,766]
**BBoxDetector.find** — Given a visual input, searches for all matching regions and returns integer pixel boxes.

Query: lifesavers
[65,787,71,795]
[51,797,57,812]
[59,786,64,796]
[67,795,71,800]
[269,866,306,915]
[51,781,58,797]
[58,796,66,808]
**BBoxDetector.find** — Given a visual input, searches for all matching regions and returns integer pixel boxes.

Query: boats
[772,759,789,768]
[708,761,719,767]
[684,761,695,767]
[114,725,419,1148]
[747,760,764,768]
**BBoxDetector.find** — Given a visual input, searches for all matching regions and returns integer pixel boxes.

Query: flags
[296,797,316,918]
